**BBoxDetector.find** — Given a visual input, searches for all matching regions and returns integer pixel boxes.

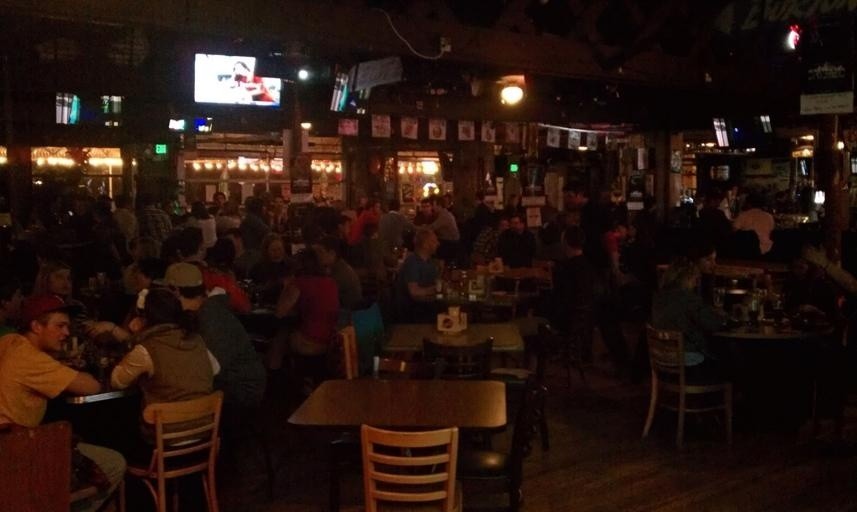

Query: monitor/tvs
[194,53,284,106]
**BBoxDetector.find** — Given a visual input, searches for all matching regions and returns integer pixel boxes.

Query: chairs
[125,389,224,510]
[361,425,462,509]
[640,325,733,452]
[339,326,358,382]
[514,267,552,290]
[70,485,99,503]
[490,324,561,386]
[464,387,545,510]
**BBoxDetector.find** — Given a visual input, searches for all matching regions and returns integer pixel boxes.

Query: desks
[384,322,522,352]
[67,392,124,403]
[714,325,797,339]
[711,263,773,279]
[443,291,541,307]
[288,380,507,428]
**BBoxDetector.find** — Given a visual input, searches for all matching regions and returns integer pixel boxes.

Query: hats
[20,293,83,324]
[153,263,202,288]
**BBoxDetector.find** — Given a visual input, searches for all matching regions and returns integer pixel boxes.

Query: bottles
[712,280,782,326]
[437,306,466,332]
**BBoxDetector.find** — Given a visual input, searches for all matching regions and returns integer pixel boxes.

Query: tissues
[437,307,467,332]
